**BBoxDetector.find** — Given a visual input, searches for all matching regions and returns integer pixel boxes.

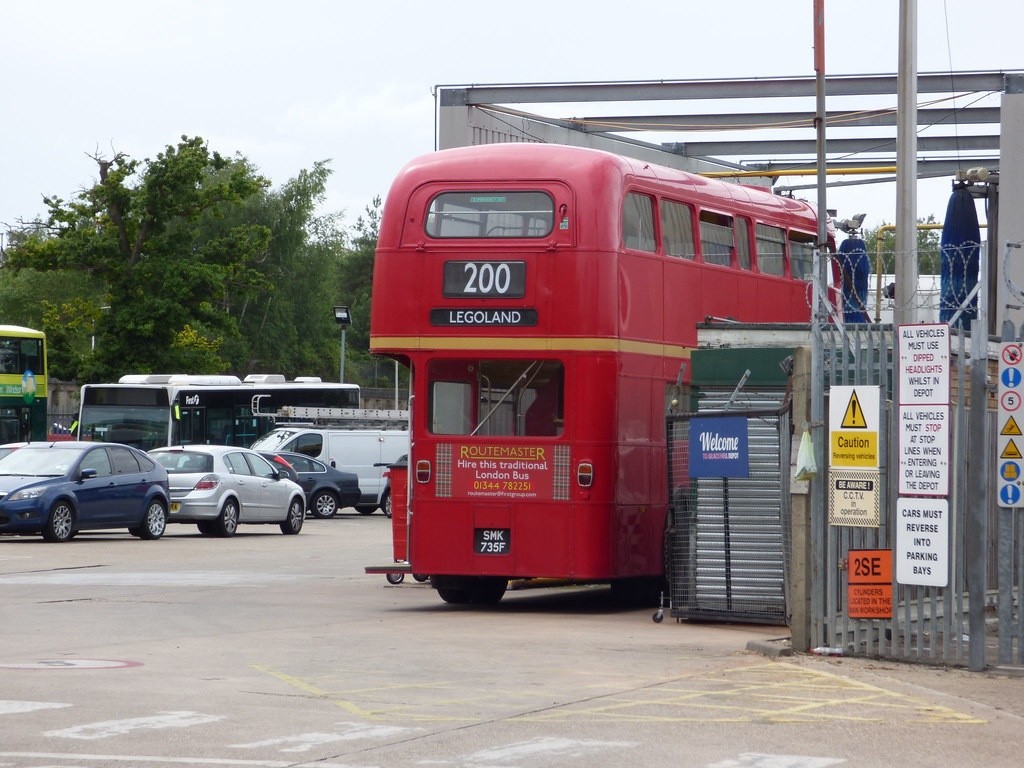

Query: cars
[248,450,363,519]
[0,440,172,543]
[145,444,308,537]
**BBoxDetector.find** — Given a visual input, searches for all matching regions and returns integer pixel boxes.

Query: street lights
[333,305,352,383]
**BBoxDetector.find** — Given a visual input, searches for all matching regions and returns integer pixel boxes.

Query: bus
[369,141,850,609]
[0,325,49,447]
[77,374,364,470]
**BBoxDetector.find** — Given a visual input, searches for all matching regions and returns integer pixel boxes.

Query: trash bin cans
[373,463,408,560]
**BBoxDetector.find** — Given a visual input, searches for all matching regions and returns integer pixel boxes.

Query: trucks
[246,395,410,519]
